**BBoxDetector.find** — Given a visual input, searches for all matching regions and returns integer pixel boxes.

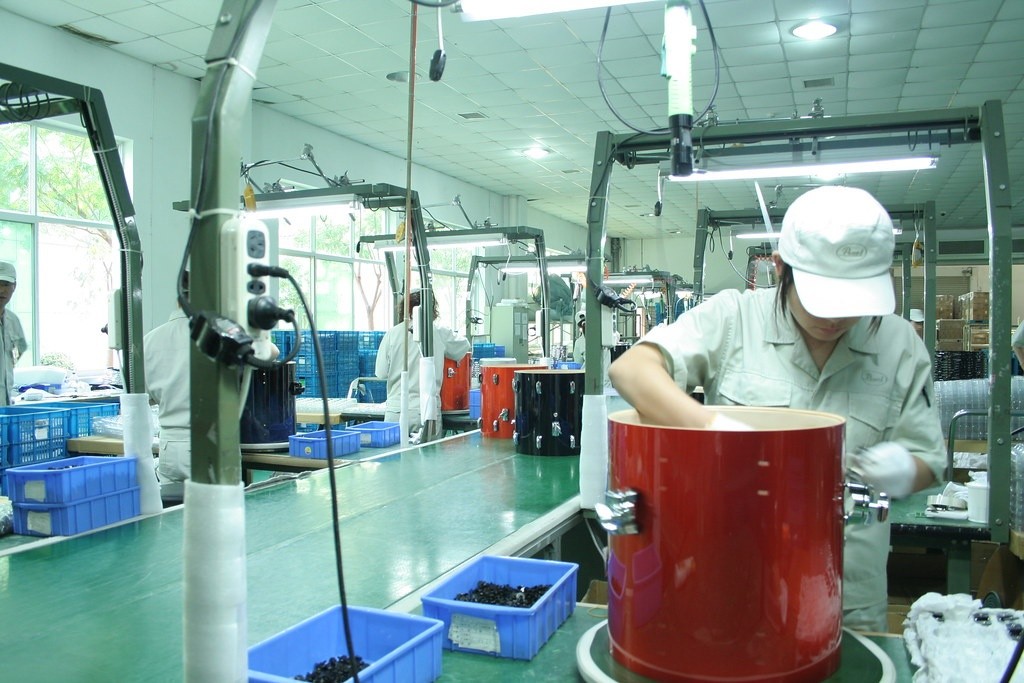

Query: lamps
[497,261,589,273]
[374,233,508,252]
[731,218,904,238]
[660,143,941,181]
[603,274,653,284]
[239,193,363,220]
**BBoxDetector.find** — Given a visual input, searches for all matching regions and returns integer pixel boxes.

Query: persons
[374,290,470,438]
[573,310,620,388]
[607,186,947,633]
[0,262,28,405]
[902,309,924,332]
[143,270,280,508]
[1011,320,1024,371]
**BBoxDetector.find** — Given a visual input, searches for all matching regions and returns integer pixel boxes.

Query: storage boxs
[296,420,362,432]
[6,456,141,537]
[472,343,505,360]
[21,384,61,393]
[357,380,388,404]
[346,421,400,447]
[580,579,608,605]
[0,402,120,497]
[288,430,361,460]
[271,331,386,398]
[422,555,579,660]
[247,604,445,683]
[557,361,582,370]
[936,292,989,352]
[469,389,482,420]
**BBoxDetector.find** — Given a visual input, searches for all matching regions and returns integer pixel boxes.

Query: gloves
[707,412,758,431]
[863,441,916,499]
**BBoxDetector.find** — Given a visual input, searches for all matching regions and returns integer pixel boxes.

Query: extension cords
[536,310,542,337]
[413,306,422,342]
[108,290,122,350]
[221,218,272,371]
[602,304,617,348]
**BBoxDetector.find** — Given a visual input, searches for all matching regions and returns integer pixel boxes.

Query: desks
[943,439,988,454]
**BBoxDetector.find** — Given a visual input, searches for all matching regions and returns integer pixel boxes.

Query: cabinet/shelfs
[484,306,528,364]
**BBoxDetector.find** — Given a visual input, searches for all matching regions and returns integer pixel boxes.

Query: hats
[575,310,586,324]
[0,261,17,282]
[780,185,896,318]
[910,308,924,323]
